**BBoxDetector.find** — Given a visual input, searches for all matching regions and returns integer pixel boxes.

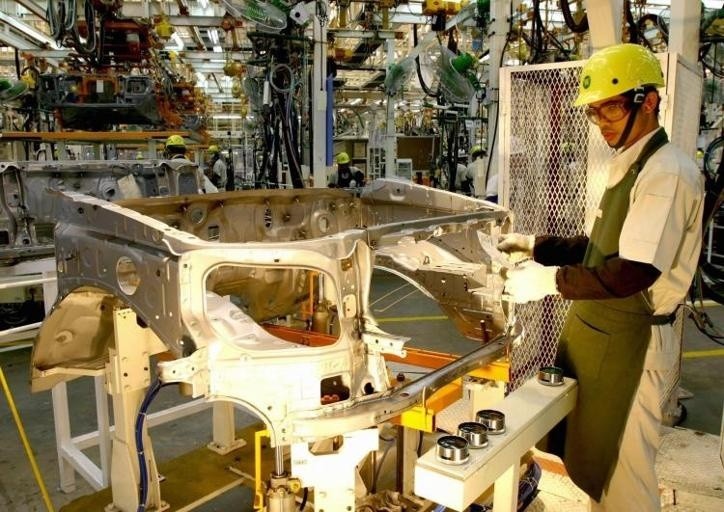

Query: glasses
[584,101,632,126]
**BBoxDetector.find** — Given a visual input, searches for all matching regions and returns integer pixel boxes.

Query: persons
[207,144,228,193]
[327,152,365,188]
[494,43,706,511]
[165,135,219,194]
[455,135,587,224]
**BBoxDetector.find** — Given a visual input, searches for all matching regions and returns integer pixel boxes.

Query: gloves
[498,232,536,258]
[503,260,560,305]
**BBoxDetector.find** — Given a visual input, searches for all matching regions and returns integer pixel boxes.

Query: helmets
[471,146,484,153]
[166,134,186,146]
[337,152,350,164]
[208,146,218,152]
[572,44,666,107]
[561,143,577,155]
